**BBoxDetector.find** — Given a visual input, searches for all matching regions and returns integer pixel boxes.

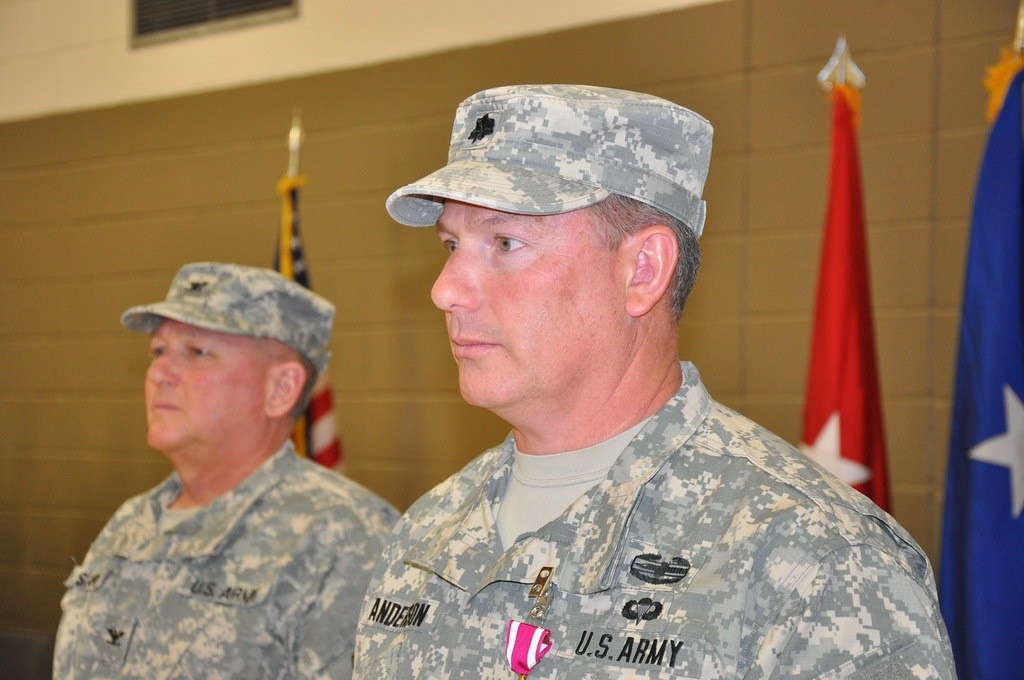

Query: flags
[936,63,1023,676]
[271,174,344,472]
[793,82,890,508]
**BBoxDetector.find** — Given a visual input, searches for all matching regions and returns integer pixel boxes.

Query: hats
[384,83,714,241]
[121,261,337,374]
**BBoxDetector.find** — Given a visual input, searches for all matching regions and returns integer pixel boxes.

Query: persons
[49,261,403,679]
[344,82,958,677]
